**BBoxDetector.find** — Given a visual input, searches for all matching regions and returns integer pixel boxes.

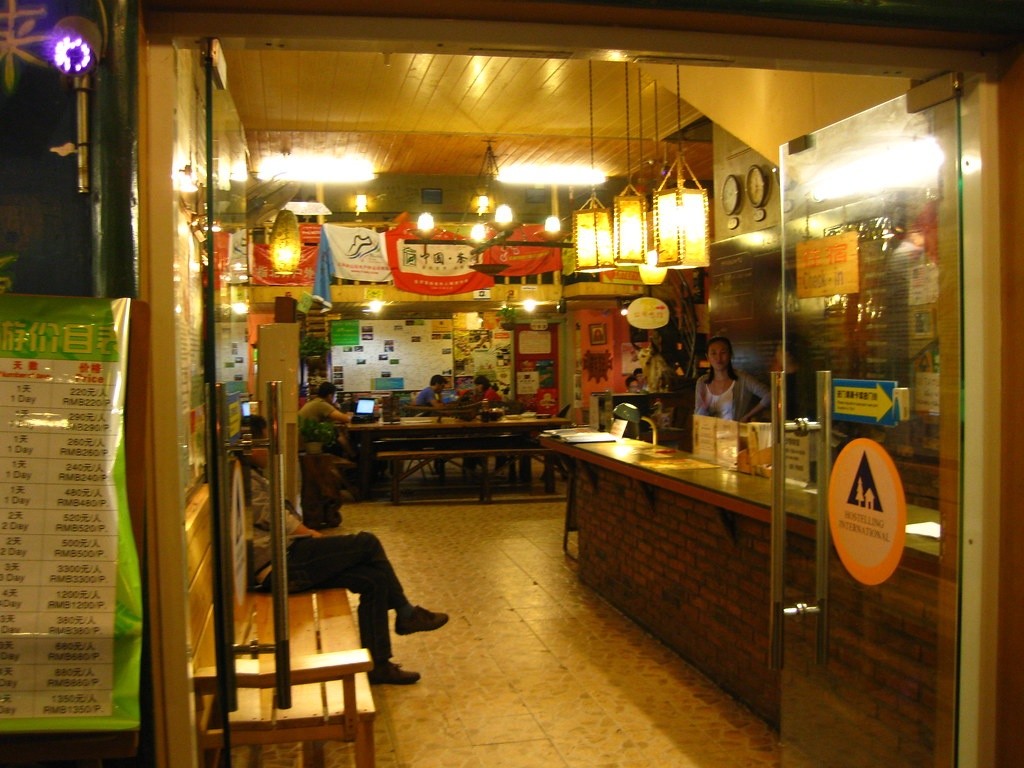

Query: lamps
[50,16,101,75]
[653,64,713,270]
[638,80,666,284]
[614,404,657,446]
[611,62,647,266]
[572,60,617,273]
[269,206,301,274]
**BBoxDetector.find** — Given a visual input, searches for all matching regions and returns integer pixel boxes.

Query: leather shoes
[394,605,448,636]
[368,662,421,685]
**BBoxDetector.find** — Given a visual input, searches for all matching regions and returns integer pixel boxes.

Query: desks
[298,453,347,530]
[334,413,571,501]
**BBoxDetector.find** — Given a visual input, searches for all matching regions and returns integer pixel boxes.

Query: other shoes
[340,490,354,501]
[494,455,509,473]
[436,476,448,482]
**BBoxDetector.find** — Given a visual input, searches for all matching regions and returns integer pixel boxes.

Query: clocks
[744,165,767,208]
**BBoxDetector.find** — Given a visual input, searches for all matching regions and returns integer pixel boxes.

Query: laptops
[355,398,376,416]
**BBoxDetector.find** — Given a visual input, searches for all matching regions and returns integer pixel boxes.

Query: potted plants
[496,305,516,331]
[298,416,336,455]
[300,334,330,375]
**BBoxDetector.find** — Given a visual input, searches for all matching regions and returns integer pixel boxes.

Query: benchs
[185,484,378,768]
[373,436,553,502]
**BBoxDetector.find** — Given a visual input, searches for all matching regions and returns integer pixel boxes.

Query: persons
[298,381,360,496]
[625,368,647,392]
[458,376,503,402]
[336,393,356,413]
[694,336,771,422]
[242,414,449,684]
[593,329,604,340]
[409,375,460,480]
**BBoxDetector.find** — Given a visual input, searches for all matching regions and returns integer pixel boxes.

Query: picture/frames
[589,324,607,345]
